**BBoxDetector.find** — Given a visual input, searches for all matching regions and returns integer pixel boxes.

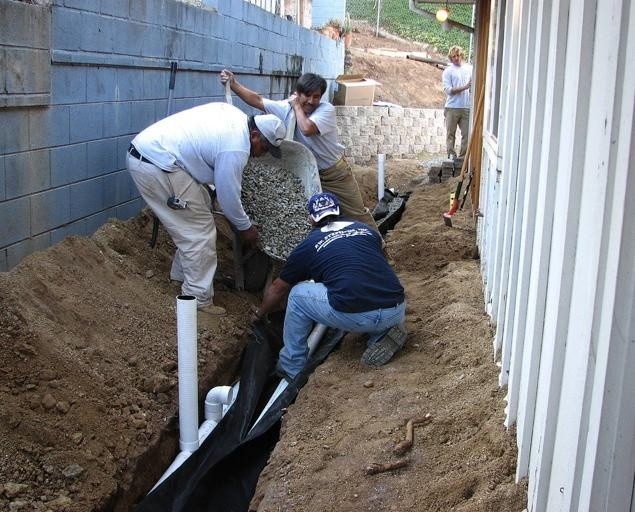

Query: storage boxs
[335,70,384,106]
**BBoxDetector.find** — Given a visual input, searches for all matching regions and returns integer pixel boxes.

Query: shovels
[442,84,484,227]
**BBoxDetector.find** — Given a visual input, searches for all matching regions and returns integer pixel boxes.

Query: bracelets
[253,309,264,320]
[464,84,468,90]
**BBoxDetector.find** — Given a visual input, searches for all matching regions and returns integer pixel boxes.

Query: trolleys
[212,77,324,291]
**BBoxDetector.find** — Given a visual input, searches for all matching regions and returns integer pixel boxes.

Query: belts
[128,144,152,164]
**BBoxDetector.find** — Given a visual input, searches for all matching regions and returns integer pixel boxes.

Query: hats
[254,114,286,158]
[308,193,340,223]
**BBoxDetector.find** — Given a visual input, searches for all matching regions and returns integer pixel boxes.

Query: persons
[441,45,471,159]
[220,67,397,268]
[125,100,288,316]
[248,192,409,383]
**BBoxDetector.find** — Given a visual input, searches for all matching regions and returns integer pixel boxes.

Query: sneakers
[276,361,294,383]
[384,246,397,270]
[361,326,408,367]
[197,304,227,317]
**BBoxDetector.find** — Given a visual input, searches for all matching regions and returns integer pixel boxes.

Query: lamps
[434,1,451,21]
